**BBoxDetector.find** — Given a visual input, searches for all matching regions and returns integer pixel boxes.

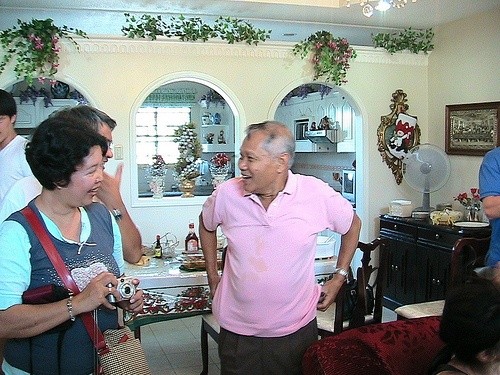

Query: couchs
[303,315,455,375]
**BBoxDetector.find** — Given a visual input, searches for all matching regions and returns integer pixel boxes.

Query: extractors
[304,130,337,154]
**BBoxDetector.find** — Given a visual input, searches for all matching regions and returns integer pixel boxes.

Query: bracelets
[66,296,78,322]
[333,268,350,283]
[112,206,126,220]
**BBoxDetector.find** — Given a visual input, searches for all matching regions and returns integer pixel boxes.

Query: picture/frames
[445,102,500,156]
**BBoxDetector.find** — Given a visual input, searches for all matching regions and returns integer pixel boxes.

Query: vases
[152,174,228,198]
[466,205,483,222]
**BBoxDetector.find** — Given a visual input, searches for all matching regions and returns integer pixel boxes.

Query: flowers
[280,30,357,107]
[198,89,226,110]
[173,123,204,181]
[0,16,89,107]
[453,188,480,220]
[144,154,168,176]
[209,152,230,175]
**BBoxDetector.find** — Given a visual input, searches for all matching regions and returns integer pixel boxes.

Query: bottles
[154,235,162,258]
[184,223,199,251]
[202,111,222,126]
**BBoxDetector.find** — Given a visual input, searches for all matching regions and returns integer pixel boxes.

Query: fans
[401,143,451,219]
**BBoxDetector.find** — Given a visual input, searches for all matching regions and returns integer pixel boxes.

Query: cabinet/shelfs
[11,97,79,128]
[380,213,493,312]
[200,100,235,152]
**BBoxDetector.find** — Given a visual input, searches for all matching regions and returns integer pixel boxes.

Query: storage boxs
[389,200,411,217]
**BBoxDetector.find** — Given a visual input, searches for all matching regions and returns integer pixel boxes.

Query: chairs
[201,238,385,375]
[394,236,490,321]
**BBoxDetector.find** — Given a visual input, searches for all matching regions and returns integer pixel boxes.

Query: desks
[120,250,339,342]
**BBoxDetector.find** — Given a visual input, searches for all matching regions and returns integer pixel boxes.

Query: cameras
[107,276,136,303]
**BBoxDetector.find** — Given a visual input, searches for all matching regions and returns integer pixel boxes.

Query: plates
[453,221,490,228]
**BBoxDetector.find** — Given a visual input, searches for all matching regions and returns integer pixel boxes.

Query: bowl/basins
[411,211,429,220]
[133,255,152,266]
[216,236,225,249]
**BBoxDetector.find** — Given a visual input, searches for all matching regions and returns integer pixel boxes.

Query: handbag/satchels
[343,279,374,321]
[98,326,152,375]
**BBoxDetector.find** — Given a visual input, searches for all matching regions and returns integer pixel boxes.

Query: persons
[478,145,500,266]
[429,265,500,375]
[0,104,143,264]
[198,120,362,375]
[0,116,145,375]
[0,89,33,210]
